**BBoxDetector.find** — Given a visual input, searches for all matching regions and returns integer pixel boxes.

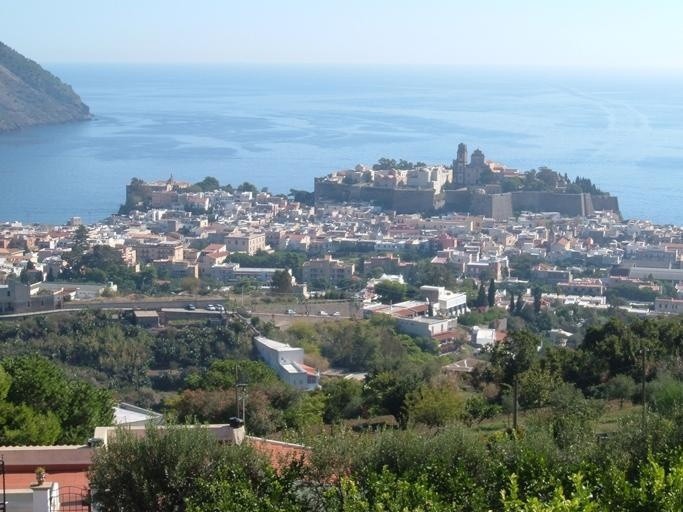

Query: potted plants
[35,467,47,485]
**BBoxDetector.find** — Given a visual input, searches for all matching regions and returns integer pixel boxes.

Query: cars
[285,309,297,314]
[317,310,341,317]
[187,303,197,311]
[205,303,225,312]
